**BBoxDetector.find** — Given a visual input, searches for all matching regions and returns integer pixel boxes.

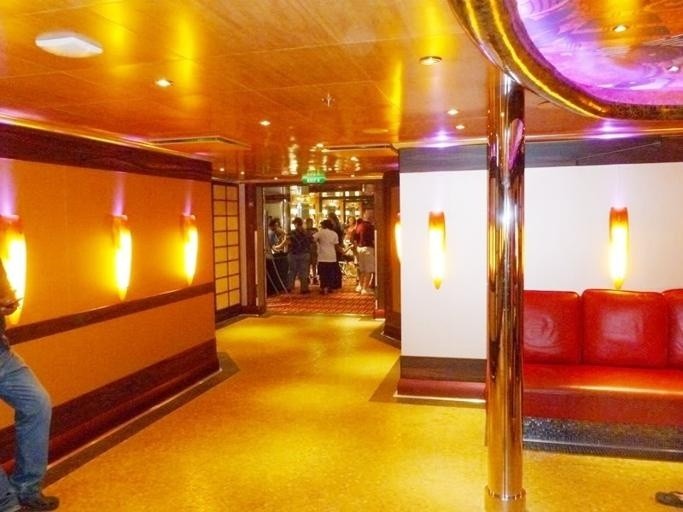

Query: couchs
[482,287,683,464]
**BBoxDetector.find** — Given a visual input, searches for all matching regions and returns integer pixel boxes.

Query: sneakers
[354,280,376,296]
[15,491,58,512]
[287,276,337,295]
[656,492,683,506]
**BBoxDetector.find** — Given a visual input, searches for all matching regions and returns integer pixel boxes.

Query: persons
[1,258,59,512]
[267,207,376,297]
